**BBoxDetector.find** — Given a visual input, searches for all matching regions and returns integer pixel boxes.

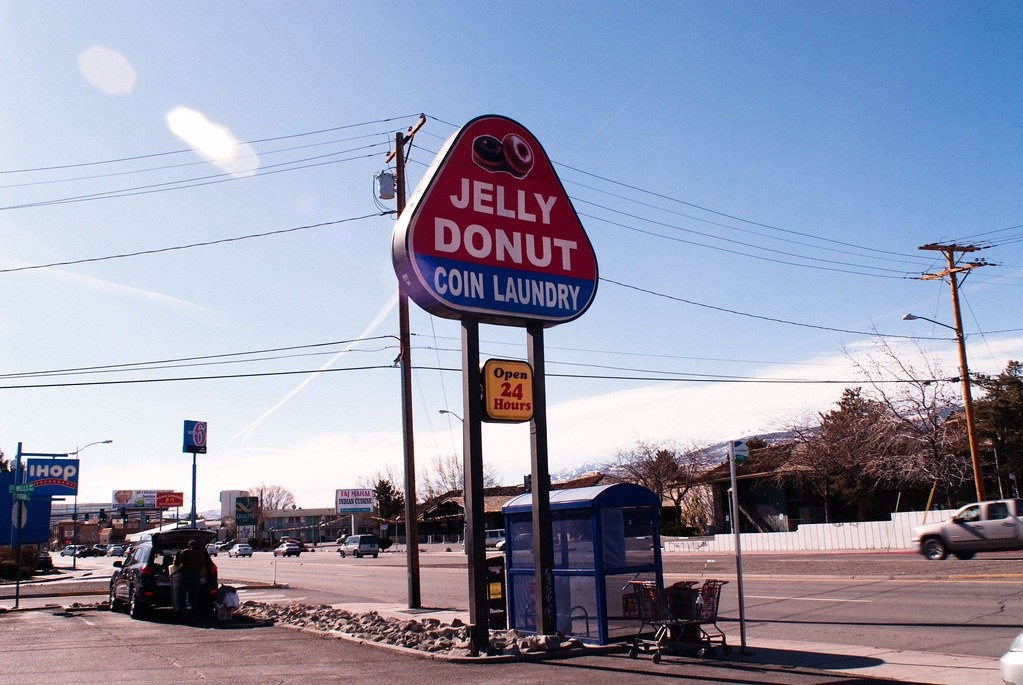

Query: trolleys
[623,577,732,664]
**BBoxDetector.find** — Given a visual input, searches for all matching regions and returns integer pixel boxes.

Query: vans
[484,529,507,547]
[340,534,380,558]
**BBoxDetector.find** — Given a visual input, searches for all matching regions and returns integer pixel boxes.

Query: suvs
[109,528,219,620]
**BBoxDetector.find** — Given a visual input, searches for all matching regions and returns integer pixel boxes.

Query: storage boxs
[217,608,233,621]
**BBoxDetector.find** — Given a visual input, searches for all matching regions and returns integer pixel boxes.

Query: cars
[206,541,232,557]
[228,544,253,558]
[60,543,132,558]
[274,536,304,557]
[336,532,352,543]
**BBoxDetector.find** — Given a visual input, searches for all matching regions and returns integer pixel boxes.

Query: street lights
[437,409,466,547]
[902,311,986,506]
[68,440,113,569]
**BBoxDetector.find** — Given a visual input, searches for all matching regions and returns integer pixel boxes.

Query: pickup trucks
[912,497,1023,559]
